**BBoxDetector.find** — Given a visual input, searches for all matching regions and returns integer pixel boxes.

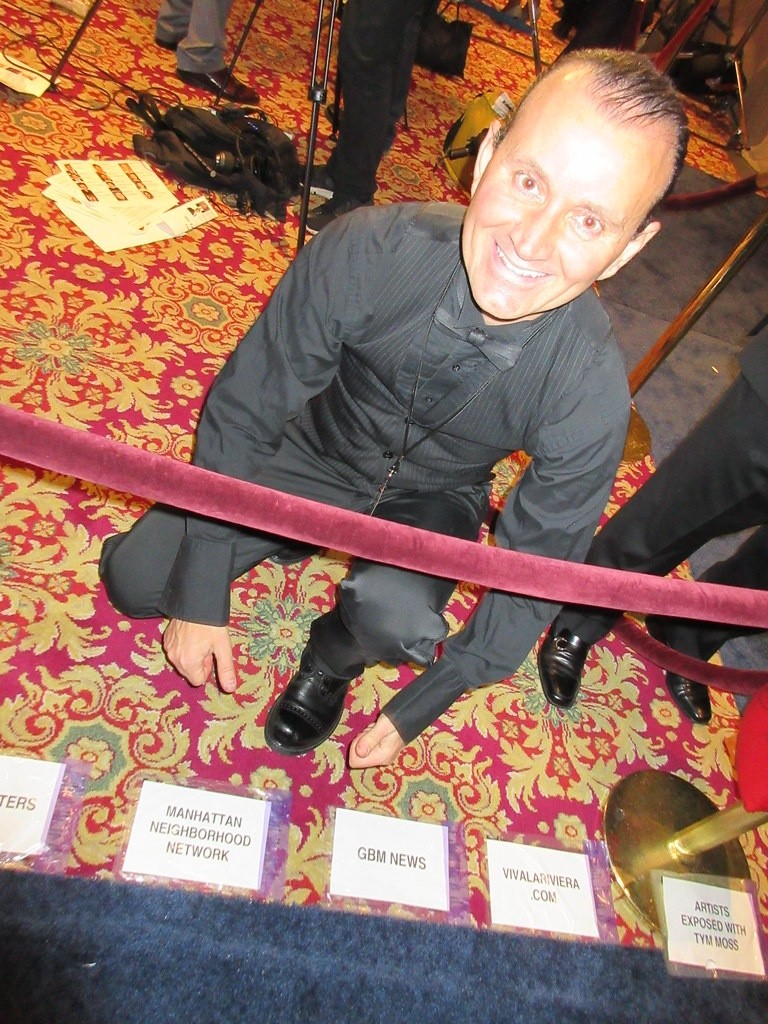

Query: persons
[98,52,691,769]
[298,1,442,236]
[188,203,209,215]
[155,1,261,103]
[119,164,154,199]
[93,164,128,202]
[64,163,98,201]
[539,323,768,723]
[7,67,35,81]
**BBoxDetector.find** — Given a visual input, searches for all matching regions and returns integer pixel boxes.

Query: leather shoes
[175,67,260,104]
[537,616,592,709]
[666,670,713,723]
[264,644,351,755]
[155,38,176,53]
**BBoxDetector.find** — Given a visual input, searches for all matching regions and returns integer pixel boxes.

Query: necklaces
[382,260,563,480]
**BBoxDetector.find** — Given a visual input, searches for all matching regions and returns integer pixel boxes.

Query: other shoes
[306,197,346,235]
[298,164,336,199]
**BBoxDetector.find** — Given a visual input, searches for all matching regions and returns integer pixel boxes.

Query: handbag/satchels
[414,0,475,78]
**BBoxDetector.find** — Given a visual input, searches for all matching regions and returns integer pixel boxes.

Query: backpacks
[126,93,302,223]
